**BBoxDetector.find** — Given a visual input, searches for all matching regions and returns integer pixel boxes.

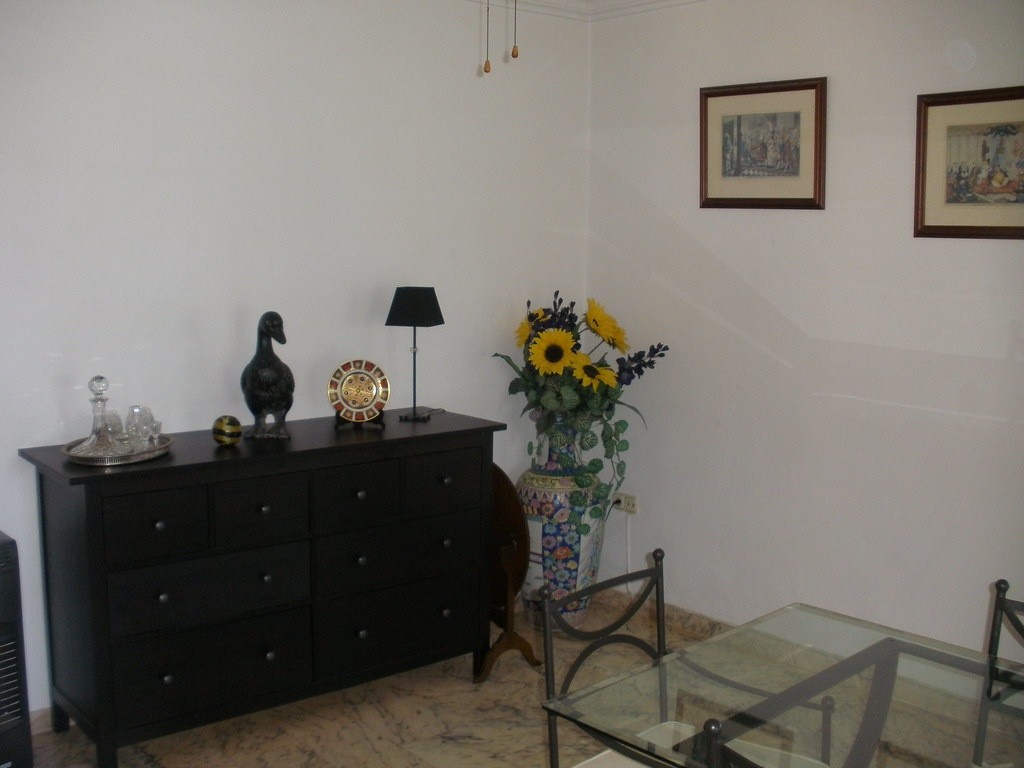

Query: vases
[514,415,607,633]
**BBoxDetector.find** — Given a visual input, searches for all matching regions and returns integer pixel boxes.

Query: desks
[540,602,1024,768]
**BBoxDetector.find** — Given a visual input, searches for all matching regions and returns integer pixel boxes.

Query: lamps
[384,286,445,423]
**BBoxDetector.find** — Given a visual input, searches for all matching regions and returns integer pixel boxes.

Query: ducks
[241,311,295,440]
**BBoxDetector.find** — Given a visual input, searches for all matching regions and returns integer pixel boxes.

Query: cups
[107,405,160,450]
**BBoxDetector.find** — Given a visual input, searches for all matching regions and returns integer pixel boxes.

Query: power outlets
[612,492,638,515]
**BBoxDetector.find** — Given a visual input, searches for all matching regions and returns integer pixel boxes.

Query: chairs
[537,548,837,768]
[972,579,1024,768]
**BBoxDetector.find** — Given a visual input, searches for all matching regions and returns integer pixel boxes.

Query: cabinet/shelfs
[16,406,508,768]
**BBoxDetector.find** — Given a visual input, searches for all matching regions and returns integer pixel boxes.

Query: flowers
[495,289,670,537]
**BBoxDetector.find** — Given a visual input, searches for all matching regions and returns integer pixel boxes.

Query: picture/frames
[699,77,828,210]
[913,85,1024,239]
